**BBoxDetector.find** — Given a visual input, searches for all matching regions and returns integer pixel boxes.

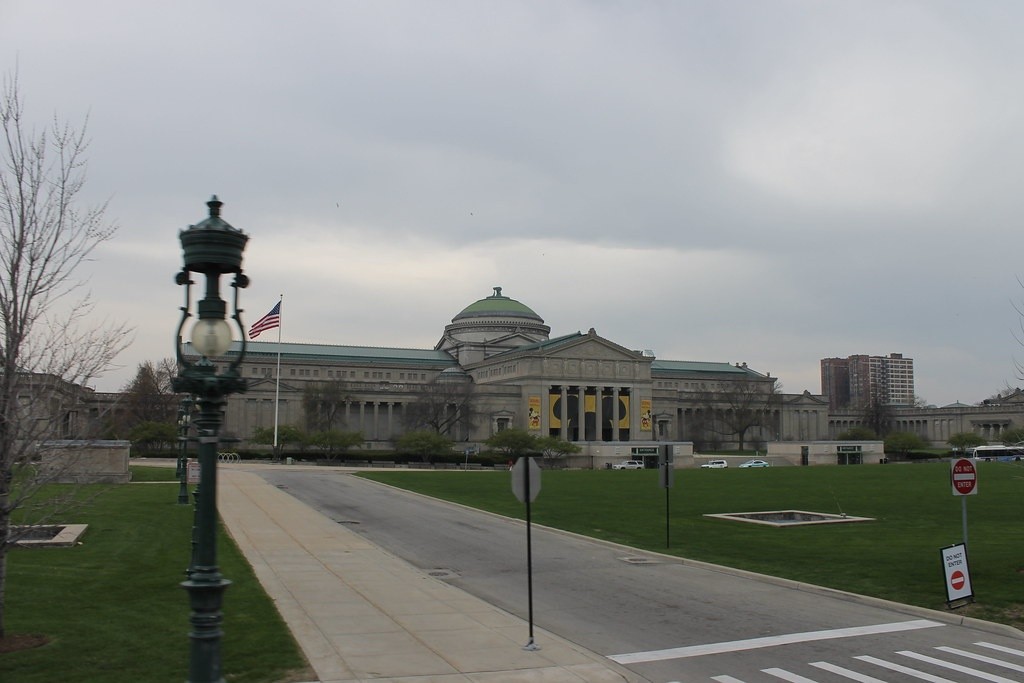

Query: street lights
[176,392,194,504]
[175,408,184,478]
[174,193,250,683]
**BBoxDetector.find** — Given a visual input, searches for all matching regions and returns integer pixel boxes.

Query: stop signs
[951,457,978,496]
[951,570,965,590]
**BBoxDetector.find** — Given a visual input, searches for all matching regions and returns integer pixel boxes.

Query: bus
[963,444,1024,462]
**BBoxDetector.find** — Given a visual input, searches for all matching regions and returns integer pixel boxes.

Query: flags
[249,301,280,340]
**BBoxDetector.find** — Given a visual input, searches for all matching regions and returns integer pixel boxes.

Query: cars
[613,460,644,470]
[700,459,728,468]
[738,459,769,468]
